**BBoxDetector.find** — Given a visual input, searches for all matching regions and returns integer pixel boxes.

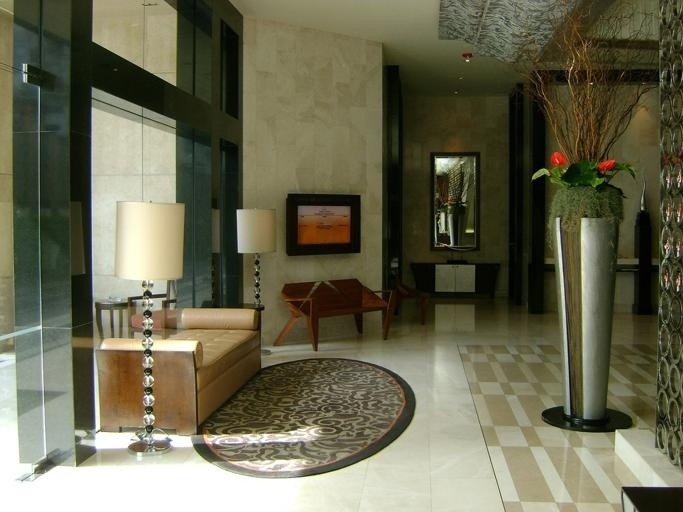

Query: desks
[94,298,136,339]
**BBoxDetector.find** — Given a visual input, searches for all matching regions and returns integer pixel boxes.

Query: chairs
[383,275,430,327]
[126,278,177,340]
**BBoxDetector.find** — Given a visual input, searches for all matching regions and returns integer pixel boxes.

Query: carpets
[190,357,415,478]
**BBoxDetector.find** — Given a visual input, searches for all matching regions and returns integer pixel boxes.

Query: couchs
[96,307,262,437]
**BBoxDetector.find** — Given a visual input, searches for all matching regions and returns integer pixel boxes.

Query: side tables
[193,303,265,326]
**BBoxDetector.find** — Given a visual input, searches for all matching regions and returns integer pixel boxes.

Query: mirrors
[429,151,481,252]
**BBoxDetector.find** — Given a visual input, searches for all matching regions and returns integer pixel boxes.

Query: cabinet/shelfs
[410,262,501,298]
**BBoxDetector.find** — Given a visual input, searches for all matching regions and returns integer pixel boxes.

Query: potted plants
[510,0,660,189]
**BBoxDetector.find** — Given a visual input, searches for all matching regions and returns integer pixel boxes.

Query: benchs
[272,278,398,351]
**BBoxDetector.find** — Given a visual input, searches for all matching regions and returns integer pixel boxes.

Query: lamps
[235,208,278,356]
[114,201,185,456]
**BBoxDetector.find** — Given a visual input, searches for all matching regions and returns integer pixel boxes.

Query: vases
[541,182,633,433]
